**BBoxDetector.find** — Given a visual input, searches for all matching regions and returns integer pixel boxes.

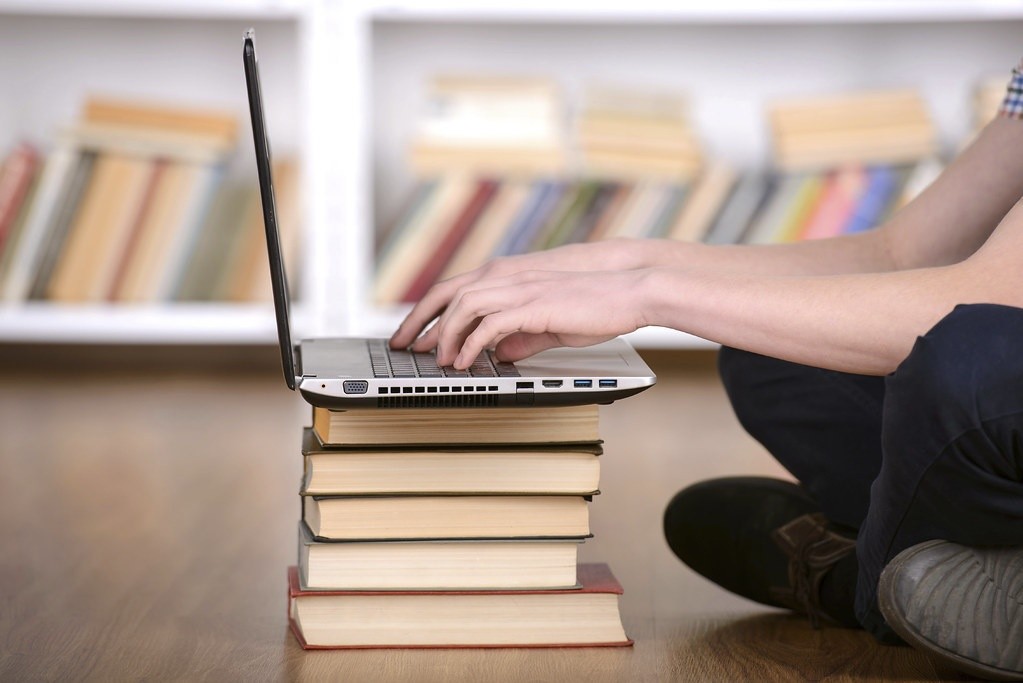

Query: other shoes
[877,539,1023,680]
[662,477,862,632]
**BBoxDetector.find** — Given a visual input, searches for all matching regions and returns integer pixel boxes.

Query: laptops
[243,29,656,414]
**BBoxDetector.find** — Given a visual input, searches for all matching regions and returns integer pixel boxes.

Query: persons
[390,60,1023,680]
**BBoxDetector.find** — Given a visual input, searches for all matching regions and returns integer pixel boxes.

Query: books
[376,77,945,306]
[296,519,583,591]
[2,100,296,304]
[288,563,634,649]
[312,406,604,448]
[298,427,601,495]
[301,494,595,543]
